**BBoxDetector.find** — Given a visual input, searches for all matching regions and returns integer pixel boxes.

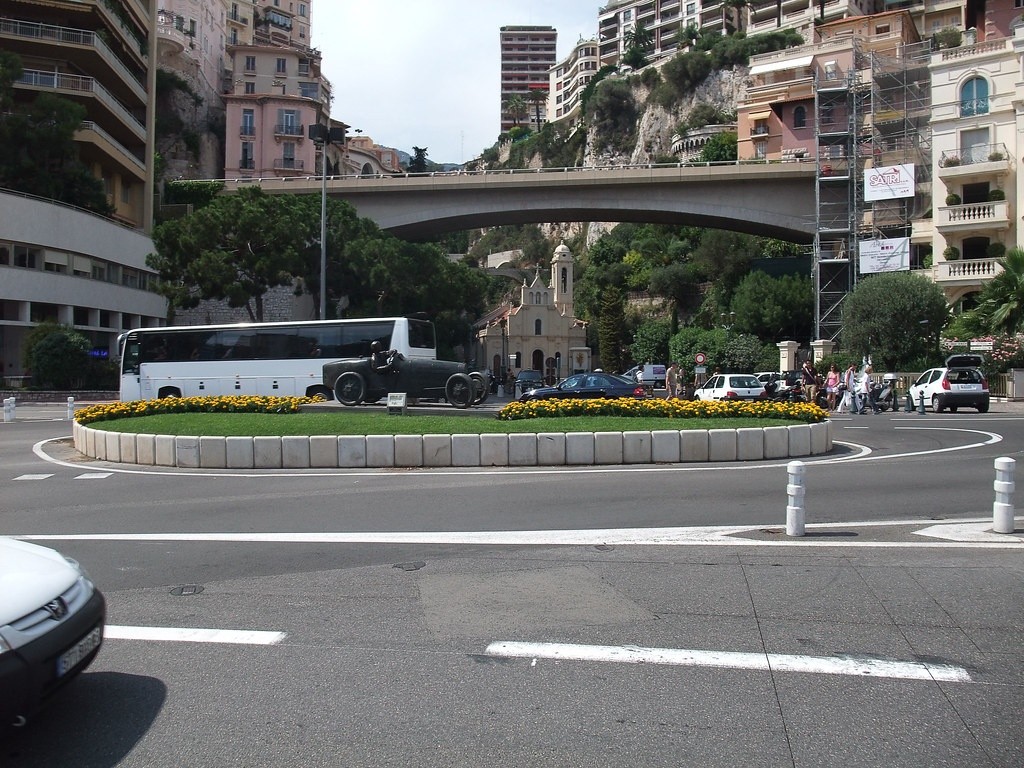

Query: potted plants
[988,190,1005,213]
[946,194,961,217]
[943,246,959,270]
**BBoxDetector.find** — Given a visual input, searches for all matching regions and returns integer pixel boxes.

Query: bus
[116,312,437,405]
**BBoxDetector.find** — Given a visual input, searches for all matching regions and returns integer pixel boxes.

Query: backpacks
[632,371,642,382]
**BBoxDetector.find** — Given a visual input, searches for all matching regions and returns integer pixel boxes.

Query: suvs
[512,369,547,397]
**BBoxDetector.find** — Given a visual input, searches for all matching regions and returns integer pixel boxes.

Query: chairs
[955,372,969,381]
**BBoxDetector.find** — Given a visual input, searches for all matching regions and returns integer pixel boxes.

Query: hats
[638,365,643,367]
[672,361,678,364]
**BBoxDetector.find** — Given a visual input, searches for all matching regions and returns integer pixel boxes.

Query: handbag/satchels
[816,385,819,392]
[846,391,852,407]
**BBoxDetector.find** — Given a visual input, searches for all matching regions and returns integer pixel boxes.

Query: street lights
[307,121,345,323]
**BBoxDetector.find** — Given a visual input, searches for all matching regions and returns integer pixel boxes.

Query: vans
[619,364,667,389]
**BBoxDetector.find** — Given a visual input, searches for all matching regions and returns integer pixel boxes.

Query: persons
[713,368,722,386]
[507,368,515,387]
[803,360,882,416]
[663,362,686,401]
[369,341,398,385]
[636,364,645,384]
[158,344,323,361]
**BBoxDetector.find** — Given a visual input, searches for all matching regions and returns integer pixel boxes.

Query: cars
[518,368,656,404]
[693,373,768,404]
[746,367,898,414]
[907,354,990,415]
[0,538,108,736]
[321,349,490,410]
